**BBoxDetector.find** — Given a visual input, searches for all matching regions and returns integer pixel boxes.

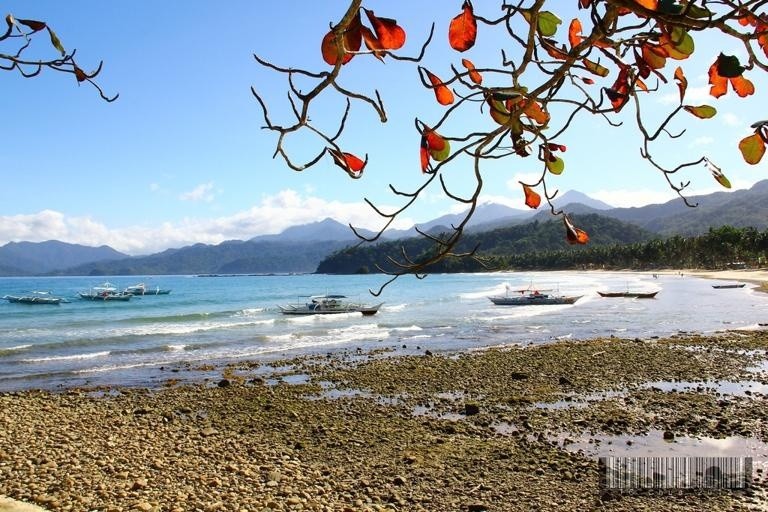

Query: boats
[597,291,658,298]
[712,284,746,288]
[276,287,385,315]
[488,281,584,304]
[1,295,60,304]
[77,282,172,300]
